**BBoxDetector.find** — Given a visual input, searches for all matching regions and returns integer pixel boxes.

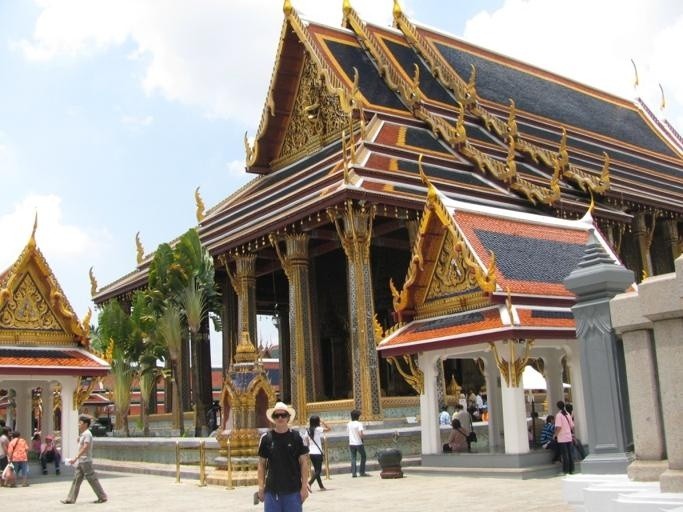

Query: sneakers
[43,469,60,475]
[307,483,326,493]
[7,481,29,487]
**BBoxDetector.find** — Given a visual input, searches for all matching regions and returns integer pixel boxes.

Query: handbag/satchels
[321,448,325,461]
[470,432,478,443]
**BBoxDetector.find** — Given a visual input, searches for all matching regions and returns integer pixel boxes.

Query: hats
[45,434,53,441]
[34,432,40,436]
[266,401,295,424]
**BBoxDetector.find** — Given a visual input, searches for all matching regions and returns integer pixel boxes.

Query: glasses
[273,413,291,418]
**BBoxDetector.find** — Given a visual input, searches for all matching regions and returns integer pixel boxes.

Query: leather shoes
[94,498,107,503]
[59,499,75,504]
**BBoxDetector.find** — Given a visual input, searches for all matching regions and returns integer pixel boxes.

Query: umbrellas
[522,365,571,405]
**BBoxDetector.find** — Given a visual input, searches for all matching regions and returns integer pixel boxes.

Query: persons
[347,410,370,477]
[0,420,60,487]
[439,388,487,452]
[258,402,309,512]
[305,415,331,492]
[60,417,107,504]
[527,401,574,475]
